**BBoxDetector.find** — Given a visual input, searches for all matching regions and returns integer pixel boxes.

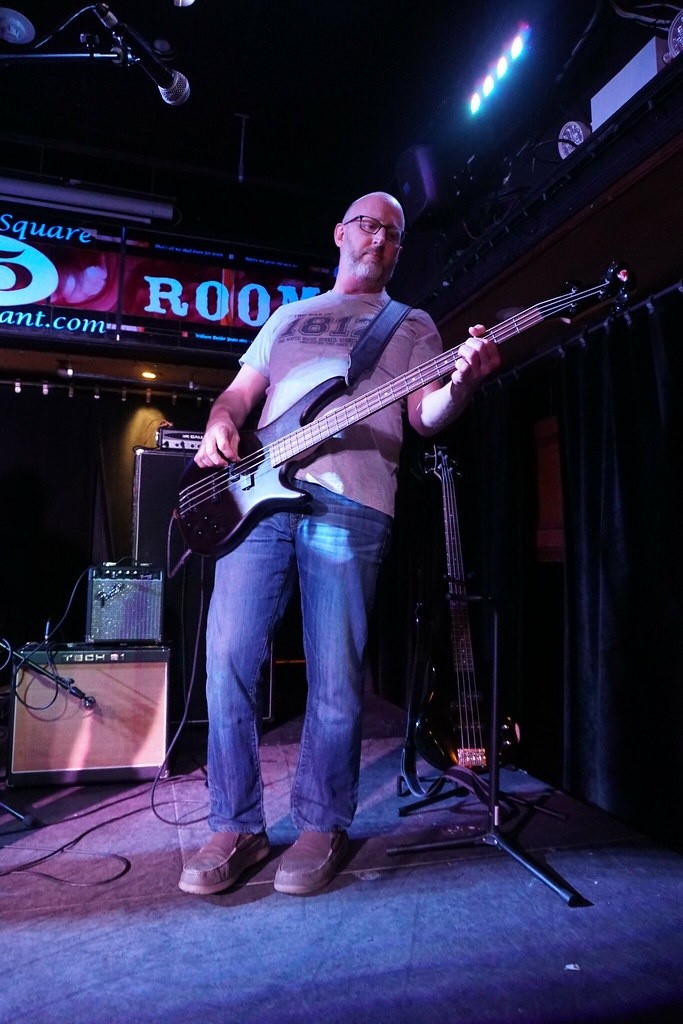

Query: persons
[176,189,503,898]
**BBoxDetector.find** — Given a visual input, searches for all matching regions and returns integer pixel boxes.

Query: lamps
[557,9,683,160]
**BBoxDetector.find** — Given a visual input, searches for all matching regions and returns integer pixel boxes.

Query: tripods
[385,575,579,907]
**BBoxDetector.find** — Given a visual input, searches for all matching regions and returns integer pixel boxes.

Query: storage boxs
[6,640,171,788]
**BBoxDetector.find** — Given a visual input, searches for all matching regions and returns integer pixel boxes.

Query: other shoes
[274,829,349,894]
[178,831,270,895]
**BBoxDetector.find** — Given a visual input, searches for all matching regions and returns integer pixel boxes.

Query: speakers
[130,445,273,721]
[395,144,445,227]
[5,645,170,787]
[84,562,166,645]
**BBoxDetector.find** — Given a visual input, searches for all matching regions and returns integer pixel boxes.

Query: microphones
[92,3,190,106]
[69,687,95,708]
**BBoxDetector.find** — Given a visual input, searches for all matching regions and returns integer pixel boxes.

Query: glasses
[343,215,408,244]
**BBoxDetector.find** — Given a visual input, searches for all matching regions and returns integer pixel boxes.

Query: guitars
[170,263,631,561]
[414,445,524,776]
